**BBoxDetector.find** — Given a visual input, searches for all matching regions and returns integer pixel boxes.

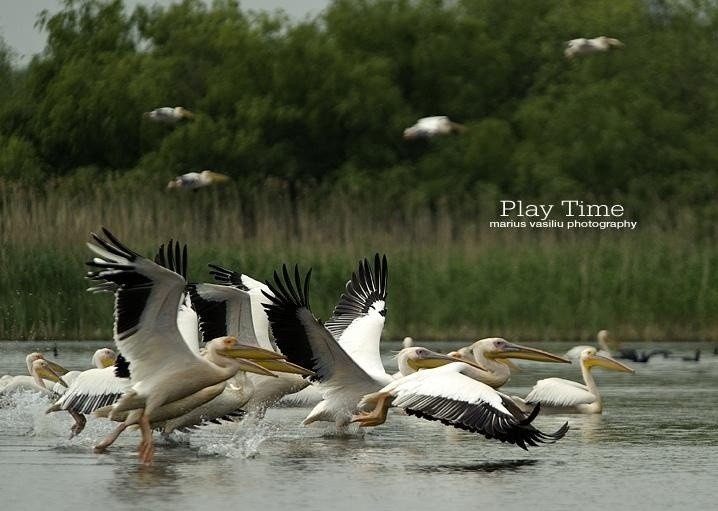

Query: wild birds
[167,169,231,190]
[0,225,637,466]
[617,346,702,363]
[564,35,625,59]
[144,105,193,127]
[402,115,461,138]
